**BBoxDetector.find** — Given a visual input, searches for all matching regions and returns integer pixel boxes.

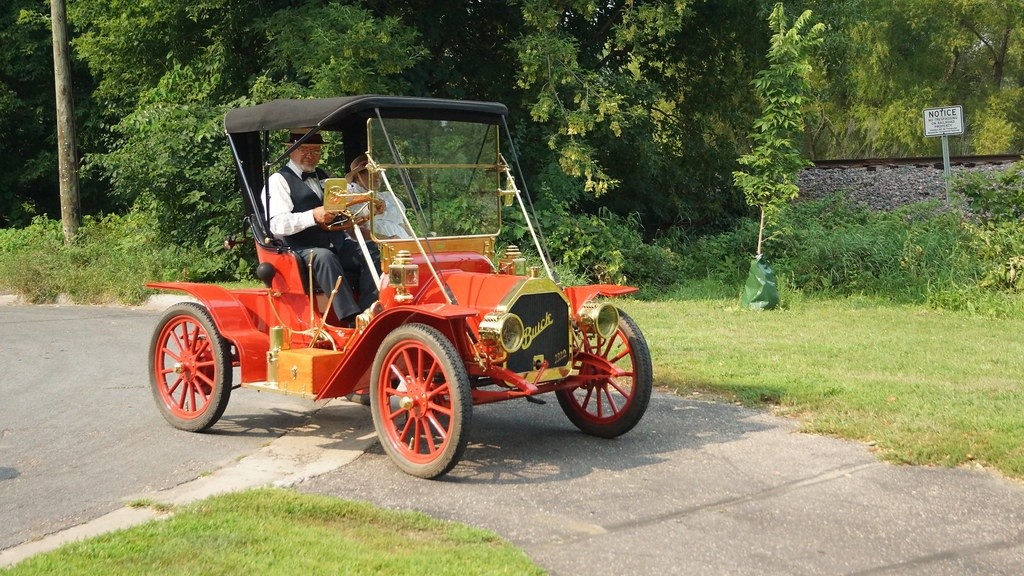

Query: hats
[346,153,380,185]
[280,128,331,147]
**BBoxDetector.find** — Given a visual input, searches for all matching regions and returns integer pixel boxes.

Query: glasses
[296,146,320,156]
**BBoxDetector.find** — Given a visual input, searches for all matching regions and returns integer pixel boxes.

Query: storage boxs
[277,348,345,394]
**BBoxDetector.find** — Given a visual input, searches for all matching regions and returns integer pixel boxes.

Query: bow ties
[301,171,317,181]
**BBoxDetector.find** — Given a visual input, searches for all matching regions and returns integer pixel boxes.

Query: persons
[261,127,382,328]
[344,156,414,242]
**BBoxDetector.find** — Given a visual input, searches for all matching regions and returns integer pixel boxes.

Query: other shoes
[348,314,358,328]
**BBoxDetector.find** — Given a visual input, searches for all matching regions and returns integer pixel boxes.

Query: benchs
[249,214,321,296]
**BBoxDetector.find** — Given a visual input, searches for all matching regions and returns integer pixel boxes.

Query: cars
[143,95,654,480]
